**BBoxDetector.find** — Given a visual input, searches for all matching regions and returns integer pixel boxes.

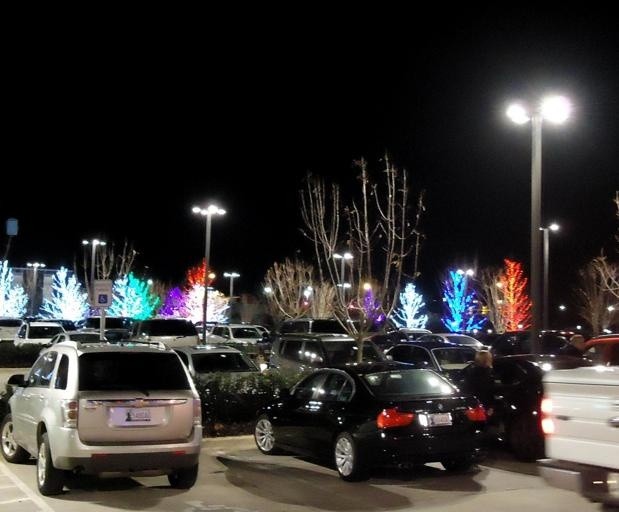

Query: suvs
[0,341,203,496]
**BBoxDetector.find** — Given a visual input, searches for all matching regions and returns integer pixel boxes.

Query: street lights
[26,261,46,317]
[82,238,105,317]
[506,92,571,360]
[191,205,226,345]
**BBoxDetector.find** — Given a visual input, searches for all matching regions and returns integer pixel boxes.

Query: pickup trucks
[541,368,619,509]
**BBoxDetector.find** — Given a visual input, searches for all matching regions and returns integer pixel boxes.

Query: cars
[253,365,488,483]
